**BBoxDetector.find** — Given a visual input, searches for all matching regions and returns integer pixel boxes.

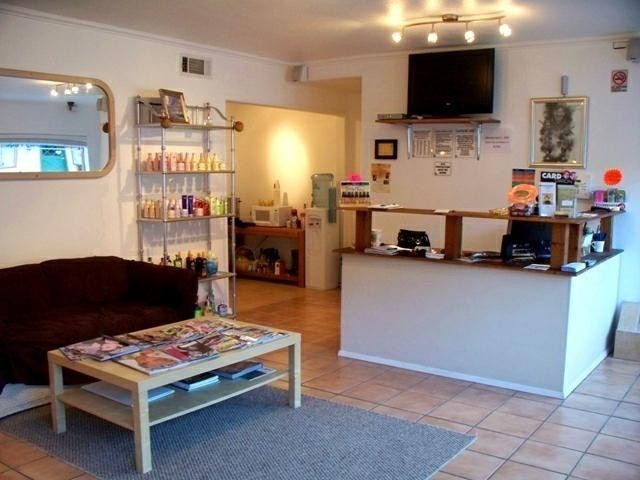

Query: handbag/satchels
[397,228,431,250]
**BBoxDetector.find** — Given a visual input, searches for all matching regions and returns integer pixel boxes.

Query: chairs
[134,93,237,321]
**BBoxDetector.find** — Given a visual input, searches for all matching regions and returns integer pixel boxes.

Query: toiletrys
[146,152,230,171]
[142,194,229,219]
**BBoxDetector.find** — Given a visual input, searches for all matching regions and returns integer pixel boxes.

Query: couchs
[0,255,200,418]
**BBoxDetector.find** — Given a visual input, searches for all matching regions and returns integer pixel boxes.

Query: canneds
[615,189,625,203]
[607,187,617,203]
[256,263,261,273]
[260,263,267,273]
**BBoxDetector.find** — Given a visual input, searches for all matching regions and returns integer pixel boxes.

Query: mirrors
[0,68,117,180]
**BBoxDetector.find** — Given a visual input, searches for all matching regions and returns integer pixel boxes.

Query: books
[58,316,290,375]
[80,360,277,407]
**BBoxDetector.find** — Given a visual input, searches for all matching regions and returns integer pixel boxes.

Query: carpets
[0,384,478,480]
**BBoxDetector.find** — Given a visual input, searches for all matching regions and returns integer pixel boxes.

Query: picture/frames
[528,96,588,170]
[374,138,398,159]
[159,88,188,123]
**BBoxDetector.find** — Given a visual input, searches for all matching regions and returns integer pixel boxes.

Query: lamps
[71,83,79,94]
[392,26,404,42]
[49,86,57,97]
[65,83,71,94]
[464,21,475,44]
[427,23,439,44]
[497,17,511,37]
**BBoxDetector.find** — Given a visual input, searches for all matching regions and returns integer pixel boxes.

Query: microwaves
[252,206,292,227]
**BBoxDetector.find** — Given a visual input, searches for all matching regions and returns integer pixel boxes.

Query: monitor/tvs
[405,46,496,117]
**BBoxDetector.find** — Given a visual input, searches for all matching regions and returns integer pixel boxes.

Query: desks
[228,222,306,287]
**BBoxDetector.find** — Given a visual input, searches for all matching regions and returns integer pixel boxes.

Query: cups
[371,228,382,248]
[591,241,605,253]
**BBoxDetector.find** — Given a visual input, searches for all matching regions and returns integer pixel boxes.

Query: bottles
[286,214,291,229]
[146,152,227,172]
[301,202,306,230]
[291,208,298,229]
[193,287,229,319]
[143,193,229,219]
[274,255,281,274]
[147,251,218,279]
